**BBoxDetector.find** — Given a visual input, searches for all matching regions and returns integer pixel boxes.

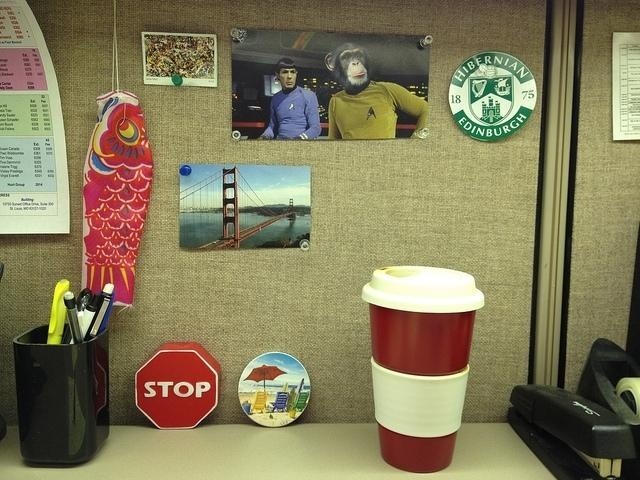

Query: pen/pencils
[48,279,115,345]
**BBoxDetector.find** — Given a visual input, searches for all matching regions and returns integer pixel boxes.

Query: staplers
[508,383,636,479]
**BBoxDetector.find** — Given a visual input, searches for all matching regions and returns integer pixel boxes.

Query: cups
[361,266,483,473]
[13,321,111,468]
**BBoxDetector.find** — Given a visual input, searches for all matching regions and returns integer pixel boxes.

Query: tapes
[575,339,638,448]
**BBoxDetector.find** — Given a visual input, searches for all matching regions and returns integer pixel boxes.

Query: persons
[321,42,429,139]
[256,57,322,140]
